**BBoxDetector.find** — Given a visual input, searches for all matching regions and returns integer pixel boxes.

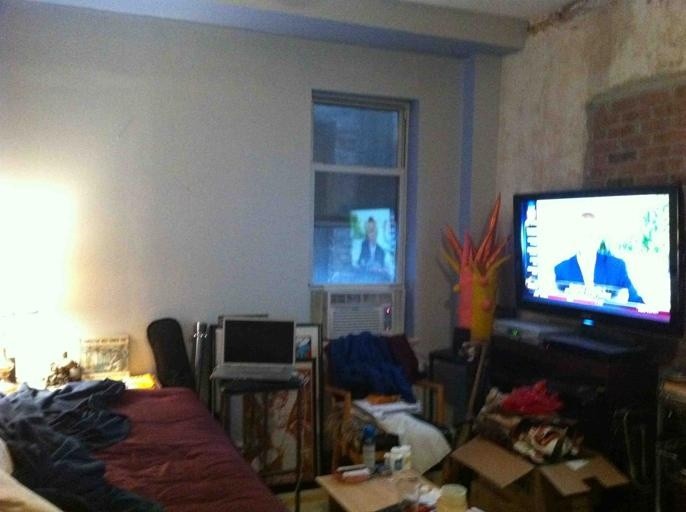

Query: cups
[393,473,421,511]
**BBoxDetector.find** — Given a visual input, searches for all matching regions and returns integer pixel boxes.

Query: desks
[655,395,686,511]
[313,462,483,512]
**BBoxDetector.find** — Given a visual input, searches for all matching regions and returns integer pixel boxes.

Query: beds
[0,387,285,509]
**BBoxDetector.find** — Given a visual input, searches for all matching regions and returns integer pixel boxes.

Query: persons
[553,213,645,304]
[357,216,385,270]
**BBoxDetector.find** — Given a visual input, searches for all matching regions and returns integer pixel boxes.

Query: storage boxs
[445,431,627,512]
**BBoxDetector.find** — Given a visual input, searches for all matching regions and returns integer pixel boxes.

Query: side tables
[429,348,487,424]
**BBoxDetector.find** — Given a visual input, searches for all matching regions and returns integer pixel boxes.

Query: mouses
[291,370,297,378]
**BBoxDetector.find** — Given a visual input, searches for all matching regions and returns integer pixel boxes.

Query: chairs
[322,339,445,473]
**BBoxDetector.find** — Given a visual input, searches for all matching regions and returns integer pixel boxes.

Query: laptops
[211,317,296,384]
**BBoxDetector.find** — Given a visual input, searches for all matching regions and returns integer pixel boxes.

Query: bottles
[438,483,468,512]
[361,439,376,467]
[385,445,414,473]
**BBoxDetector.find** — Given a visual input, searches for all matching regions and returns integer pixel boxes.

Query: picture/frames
[221,322,320,492]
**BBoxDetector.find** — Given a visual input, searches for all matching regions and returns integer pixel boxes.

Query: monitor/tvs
[347,204,396,282]
[513,184,685,333]
